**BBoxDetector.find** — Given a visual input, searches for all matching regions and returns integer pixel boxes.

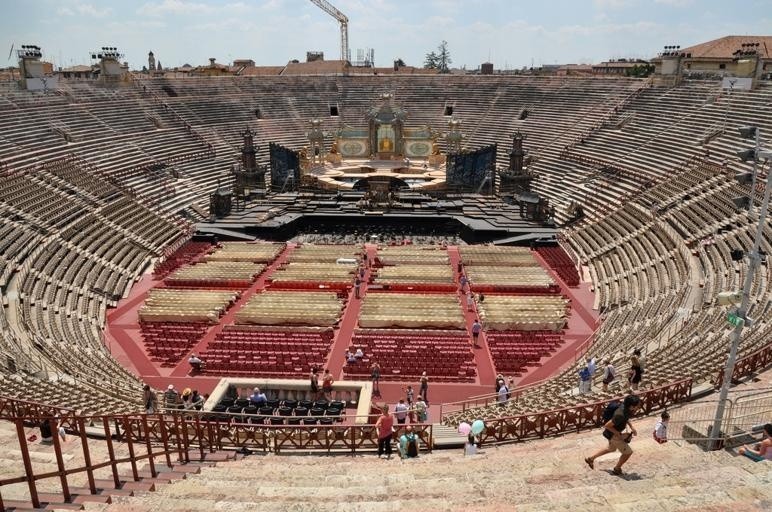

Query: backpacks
[404,433,419,457]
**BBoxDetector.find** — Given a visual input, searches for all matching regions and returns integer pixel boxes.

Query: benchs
[1,74,771,512]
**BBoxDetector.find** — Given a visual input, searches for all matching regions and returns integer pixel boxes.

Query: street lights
[101,46,121,59]
[705,126,772,458]
[738,41,760,57]
[22,45,43,57]
[663,44,682,57]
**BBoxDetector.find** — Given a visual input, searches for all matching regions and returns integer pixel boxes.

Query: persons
[652,410,671,443]
[164,384,177,407]
[457,258,486,313]
[41,414,66,442]
[180,385,209,411]
[739,423,772,460]
[354,250,369,300]
[308,346,517,461]
[188,352,201,363]
[437,200,441,215]
[472,320,481,345]
[584,394,641,474]
[577,346,646,395]
[249,387,268,402]
[302,226,460,251]
[143,384,157,412]
